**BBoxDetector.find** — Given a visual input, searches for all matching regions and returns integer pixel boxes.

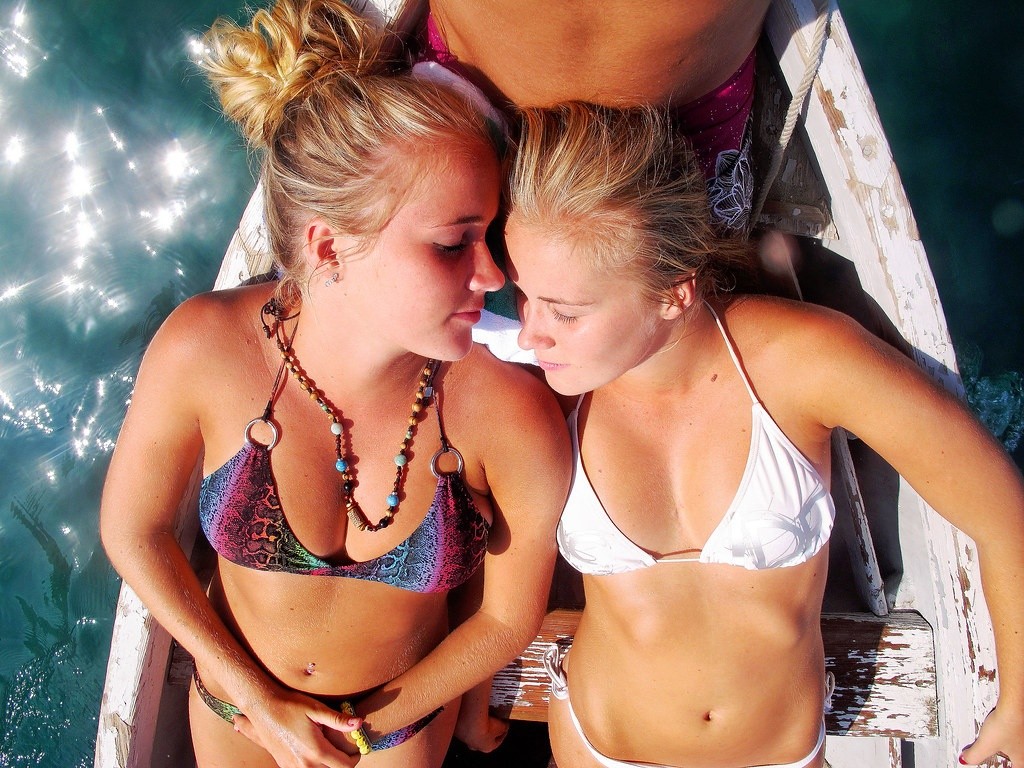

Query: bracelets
[342,702,371,755]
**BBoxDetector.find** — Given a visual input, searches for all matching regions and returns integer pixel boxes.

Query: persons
[457,98,1024,768]
[416,0,770,319]
[97,0,574,768]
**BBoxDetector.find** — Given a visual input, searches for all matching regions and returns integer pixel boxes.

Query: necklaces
[262,289,436,532]
